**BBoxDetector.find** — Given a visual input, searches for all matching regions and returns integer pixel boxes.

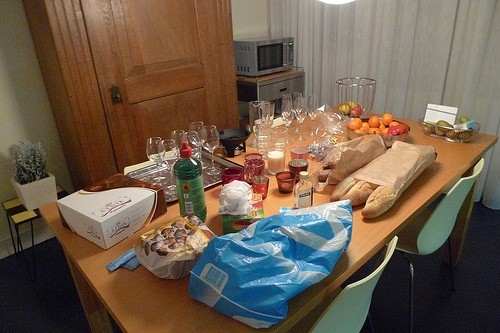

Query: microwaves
[233,35,294,76]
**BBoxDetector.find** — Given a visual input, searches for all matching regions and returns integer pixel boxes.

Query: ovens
[236,67,306,131]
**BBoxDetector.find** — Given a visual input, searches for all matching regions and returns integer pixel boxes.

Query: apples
[338,103,351,114]
[350,104,362,117]
[387,124,408,137]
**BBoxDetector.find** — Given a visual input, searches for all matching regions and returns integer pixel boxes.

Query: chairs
[384,157,485,333]
[288,234,398,333]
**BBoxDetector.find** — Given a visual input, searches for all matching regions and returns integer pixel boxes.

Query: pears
[423,115,475,139]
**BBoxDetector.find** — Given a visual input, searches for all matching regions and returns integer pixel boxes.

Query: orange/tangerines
[348,113,398,136]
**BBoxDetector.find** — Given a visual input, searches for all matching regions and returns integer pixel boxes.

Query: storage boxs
[56,172,168,250]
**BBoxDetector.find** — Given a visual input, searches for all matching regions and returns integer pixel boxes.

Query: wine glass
[248,91,318,144]
[146,121,222,176]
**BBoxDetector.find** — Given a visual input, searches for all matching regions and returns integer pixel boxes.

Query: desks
[37,107,497,333]
[0,184,70,282]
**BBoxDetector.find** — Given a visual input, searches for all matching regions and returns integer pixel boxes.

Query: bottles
[293,171,315,209]
[173,141,206,224]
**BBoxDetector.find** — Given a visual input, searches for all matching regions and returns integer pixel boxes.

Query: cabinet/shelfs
[22,0,239,191]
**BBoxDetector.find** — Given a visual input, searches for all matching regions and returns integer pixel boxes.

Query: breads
[317,148,341,183]
[330,176,398,219]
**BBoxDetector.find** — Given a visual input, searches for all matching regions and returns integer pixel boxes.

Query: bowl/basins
[218,128,250,147]
[418,114,481,143]
[347,118,410,148]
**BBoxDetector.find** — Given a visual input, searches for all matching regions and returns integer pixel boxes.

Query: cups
[334,77,376,119]
[222,124,309,200]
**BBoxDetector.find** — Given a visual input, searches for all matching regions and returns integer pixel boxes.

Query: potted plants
[6,138,58,211]
